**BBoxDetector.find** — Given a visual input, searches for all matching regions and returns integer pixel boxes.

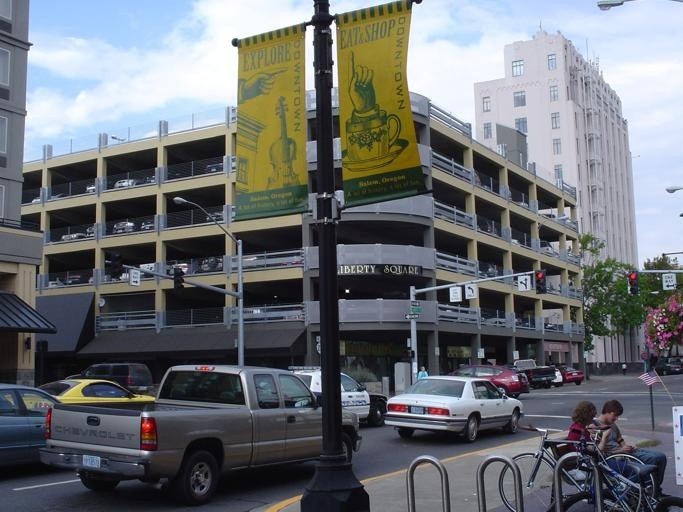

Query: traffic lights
[104,252,124,279]
[626,269,639,297]
[535,269,546,294]
[174,267,185,295]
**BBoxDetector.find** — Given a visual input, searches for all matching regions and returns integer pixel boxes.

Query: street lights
[597,0,683,11]
[173,197,244,366]
[665,185,683,193]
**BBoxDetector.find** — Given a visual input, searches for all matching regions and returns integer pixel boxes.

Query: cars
[206,162,222,173]
[540,240,553,252]
[0,384,76,472]
[544,323,557,330]
[112,219,154,234]
[64,272,82,284]
[32,197,40,203]
[167,257,223,274]
[501,359,585,392]
[206,212,223,221]
[85,183,95,192]
[486,318,506,324]
[261,366,389,427]
[61,225,94,240]
[664,357,683,375]
[382,375,525,443]
[425,365,530,398]
[515,314,534,327]
[114,175,155,188]
[479,218,497,233]
[5,378,157,414]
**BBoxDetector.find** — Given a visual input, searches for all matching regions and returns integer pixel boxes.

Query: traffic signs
[130,270,140,286]
[662,274,677,291]
[404,300,422,319]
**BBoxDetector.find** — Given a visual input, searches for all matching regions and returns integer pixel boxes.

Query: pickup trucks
[37,365,364,504]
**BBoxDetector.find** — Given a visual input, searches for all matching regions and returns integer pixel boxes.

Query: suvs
[66,363,155,394]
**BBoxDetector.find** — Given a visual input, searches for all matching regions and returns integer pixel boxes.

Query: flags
[637,369,662,387]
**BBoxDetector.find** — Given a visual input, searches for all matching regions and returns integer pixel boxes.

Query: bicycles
[498,426,656,512]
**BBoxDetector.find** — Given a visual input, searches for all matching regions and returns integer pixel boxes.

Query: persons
[417,365,427,382]
[594,359,601,376]
[586,399,673,500]
[567,400,662,511]
[621,362,627,375]
